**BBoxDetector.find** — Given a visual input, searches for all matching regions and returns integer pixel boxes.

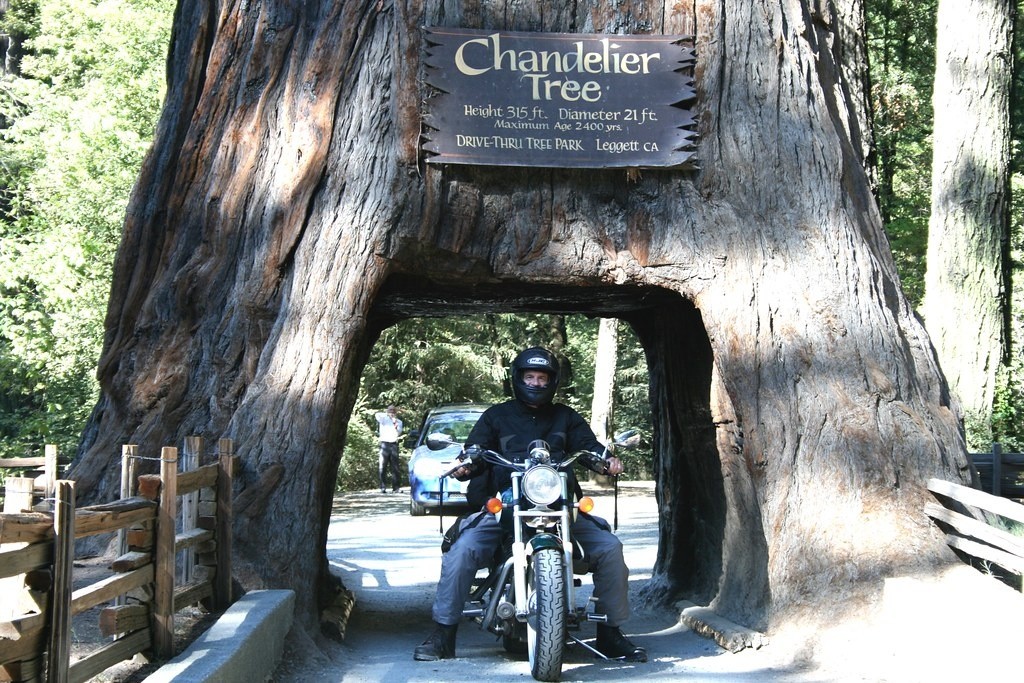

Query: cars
[403,404,493,516]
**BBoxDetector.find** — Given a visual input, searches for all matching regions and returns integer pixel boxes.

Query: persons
[413,346,649,662]
[375,405,404,494]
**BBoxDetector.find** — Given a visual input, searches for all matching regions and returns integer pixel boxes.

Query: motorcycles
[425,426,642,681]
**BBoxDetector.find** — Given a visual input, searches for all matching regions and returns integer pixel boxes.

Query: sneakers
[414,623,459,660]
[596,622,647,662]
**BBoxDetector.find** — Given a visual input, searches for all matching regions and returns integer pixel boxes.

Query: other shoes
[392,489,403,493]
[381,489,385,493]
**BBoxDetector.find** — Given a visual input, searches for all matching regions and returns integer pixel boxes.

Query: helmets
[511,348,560,405]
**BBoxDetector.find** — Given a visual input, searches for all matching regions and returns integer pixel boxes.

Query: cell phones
[392,417,396,423]
